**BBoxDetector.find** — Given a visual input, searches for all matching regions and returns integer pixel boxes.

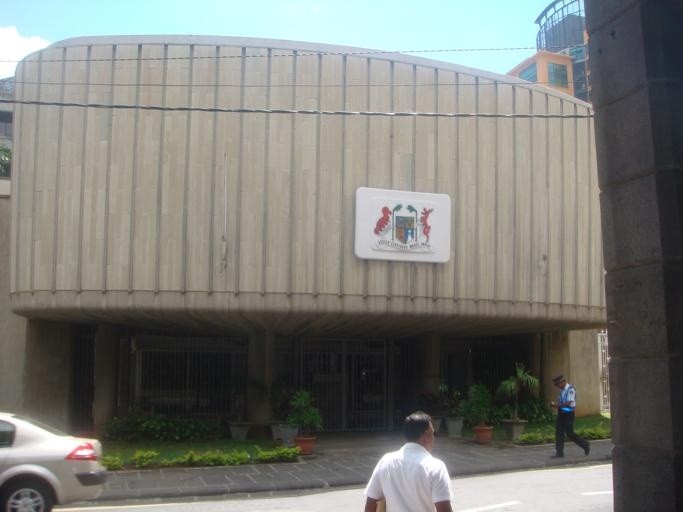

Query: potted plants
[422,361,540,444]
[222,370,325,455]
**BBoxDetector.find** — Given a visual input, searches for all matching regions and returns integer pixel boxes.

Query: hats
[552,375,565,385]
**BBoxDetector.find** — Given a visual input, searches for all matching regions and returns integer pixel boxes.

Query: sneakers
[584,442,591,455]
[551,452,564,458]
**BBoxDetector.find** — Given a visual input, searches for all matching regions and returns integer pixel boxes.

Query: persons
[364,412,453,512]
[550,374,590,459]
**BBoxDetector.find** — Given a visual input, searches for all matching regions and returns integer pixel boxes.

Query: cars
[0,412,111,511]
